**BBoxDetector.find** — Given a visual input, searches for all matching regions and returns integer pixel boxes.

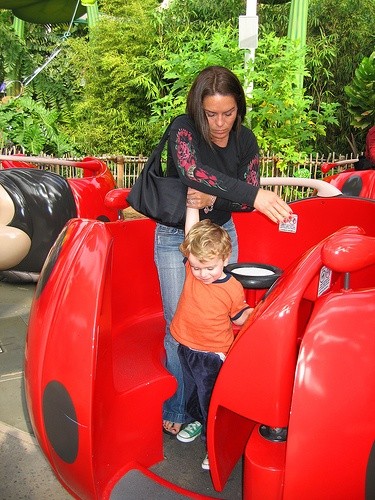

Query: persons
[176,208,253,469]
[155,65,293,436]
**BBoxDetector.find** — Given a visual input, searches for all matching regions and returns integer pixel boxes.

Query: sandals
[162,418,182,436]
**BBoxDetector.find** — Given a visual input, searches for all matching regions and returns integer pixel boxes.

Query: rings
[188,199,193,205]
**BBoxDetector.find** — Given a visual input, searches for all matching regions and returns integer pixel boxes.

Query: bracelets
[204,196,215,213]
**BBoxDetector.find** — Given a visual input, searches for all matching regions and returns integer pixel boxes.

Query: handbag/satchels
[126,114,195,228]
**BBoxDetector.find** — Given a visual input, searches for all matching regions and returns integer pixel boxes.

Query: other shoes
[202,452,209,470]
[176,421,204,443]
